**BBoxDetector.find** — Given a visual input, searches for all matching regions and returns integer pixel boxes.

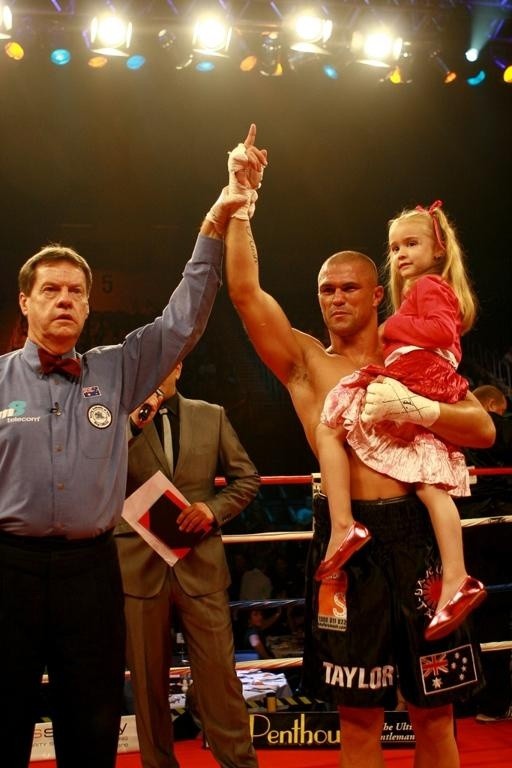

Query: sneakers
[473,706,512,722]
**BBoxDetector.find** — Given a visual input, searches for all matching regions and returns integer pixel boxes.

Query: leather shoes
[421,574,489,646]
[311,523,373,584]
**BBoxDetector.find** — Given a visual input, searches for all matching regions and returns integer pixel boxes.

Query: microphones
[138,388,164,422]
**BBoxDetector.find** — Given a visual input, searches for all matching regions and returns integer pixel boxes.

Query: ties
[155,407,179,481]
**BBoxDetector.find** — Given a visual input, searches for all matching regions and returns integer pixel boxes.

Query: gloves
[359,376,443,431]
[220,140,273,225]
[199,185,261,234]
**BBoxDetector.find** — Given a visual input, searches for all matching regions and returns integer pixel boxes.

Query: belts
[0,540,117,570]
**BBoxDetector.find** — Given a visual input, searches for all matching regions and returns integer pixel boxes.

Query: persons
[0,186,258,768]
[314,206,486,641]
[470,386,507,415]
[113,357,261,765]
[227,122,495,768]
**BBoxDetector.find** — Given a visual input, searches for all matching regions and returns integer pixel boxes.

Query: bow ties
[33,351,87,377]
